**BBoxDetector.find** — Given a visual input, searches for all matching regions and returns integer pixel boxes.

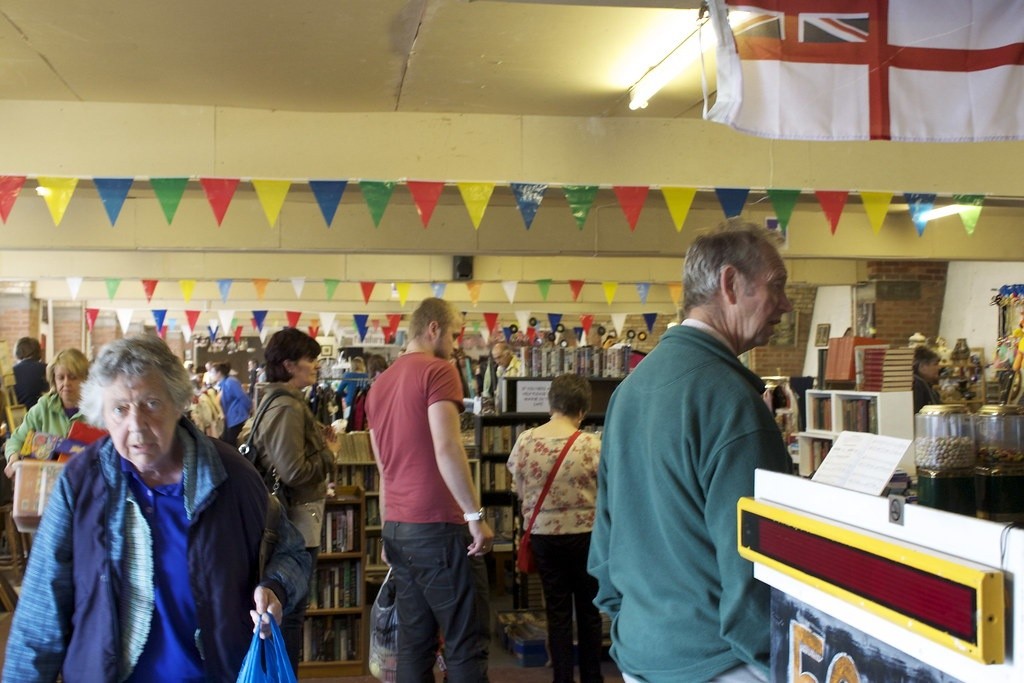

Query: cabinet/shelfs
[323,463,391,585]
[291,487,365,680]
[794,389,916,474]
[474,416,552,553]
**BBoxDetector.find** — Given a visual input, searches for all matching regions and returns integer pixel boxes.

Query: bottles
[908,332,1022,470]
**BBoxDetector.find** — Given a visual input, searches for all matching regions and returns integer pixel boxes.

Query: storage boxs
[497,609,547,666]
[10,460,67,532]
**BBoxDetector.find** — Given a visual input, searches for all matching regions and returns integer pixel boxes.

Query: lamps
[914,200,974,223]
[624,6,749,110]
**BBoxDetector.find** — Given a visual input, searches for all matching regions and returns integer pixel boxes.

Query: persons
[911,346,941,412]
[491,343,522,379]
[4,336,51,409]
[181,358,267,446]
[505,373,603,683]
[243,328,342,677]
[4,348,91,480]
[365,298,494,683]
[587,228,793,683]
[0,333,312,683]
[337,354,390,406]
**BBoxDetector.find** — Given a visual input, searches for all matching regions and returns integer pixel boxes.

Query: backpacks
[345,385,371,433]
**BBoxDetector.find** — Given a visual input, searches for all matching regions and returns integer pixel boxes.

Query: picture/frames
[815,324,830,347]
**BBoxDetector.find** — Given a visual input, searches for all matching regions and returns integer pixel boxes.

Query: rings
[482,546,486,552]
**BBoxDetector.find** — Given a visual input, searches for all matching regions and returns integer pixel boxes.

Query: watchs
[463,507,486,521]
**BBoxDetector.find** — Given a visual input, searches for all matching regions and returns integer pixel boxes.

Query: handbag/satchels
[369,566,445,683]
[236,611,297,683]
[235,389,306,521]
[516,533,538,574]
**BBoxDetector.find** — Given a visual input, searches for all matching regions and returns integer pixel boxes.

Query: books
[519,344,630,377]
[298,423,606,662]
[811,347,914,473]
